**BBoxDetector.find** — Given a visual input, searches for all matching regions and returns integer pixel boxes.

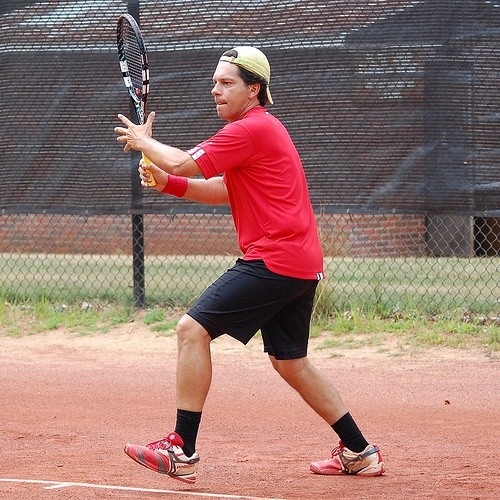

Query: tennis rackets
[116,13,159,188]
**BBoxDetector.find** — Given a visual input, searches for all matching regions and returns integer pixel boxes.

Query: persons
[114,46,386,484]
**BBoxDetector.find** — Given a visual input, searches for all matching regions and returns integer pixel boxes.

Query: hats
[220,46,273,105]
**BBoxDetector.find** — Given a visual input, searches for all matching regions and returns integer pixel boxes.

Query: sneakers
[124,432,200,484]
[310,440,385,476]
